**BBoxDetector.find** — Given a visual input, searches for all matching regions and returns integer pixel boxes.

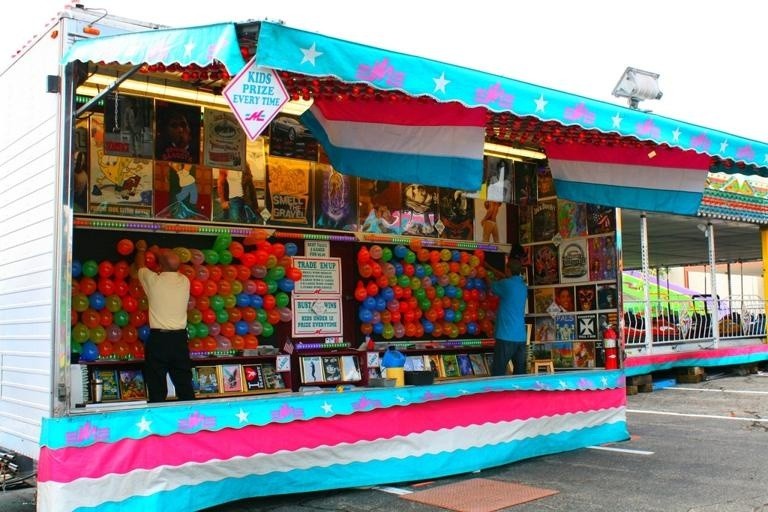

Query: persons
[489,158,512,204]
[135,238,195,401]
[598,284,617,309]
[124,98,146,159]
[155,111,200,164]
[481,200,501,243]
[556,288,570,313]
[217,161,261,225]
[477,257,528,376]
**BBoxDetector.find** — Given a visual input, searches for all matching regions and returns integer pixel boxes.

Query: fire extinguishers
[603,323,618,370]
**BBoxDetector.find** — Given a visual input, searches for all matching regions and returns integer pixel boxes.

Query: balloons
[71,240,159,362]
[176,229,301,350]
[355,239,502,348]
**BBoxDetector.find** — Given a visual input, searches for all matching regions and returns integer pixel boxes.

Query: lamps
[612,67,664,115]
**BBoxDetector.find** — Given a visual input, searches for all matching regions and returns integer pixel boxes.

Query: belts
[151,329,186,334]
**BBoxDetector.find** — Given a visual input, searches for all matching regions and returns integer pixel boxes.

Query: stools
[529,358,556,375]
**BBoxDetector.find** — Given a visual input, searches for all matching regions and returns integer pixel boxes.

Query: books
[93,370,175,403]
[298,357,361,392]
[369,352,515,380]
[190,365,285,395]
